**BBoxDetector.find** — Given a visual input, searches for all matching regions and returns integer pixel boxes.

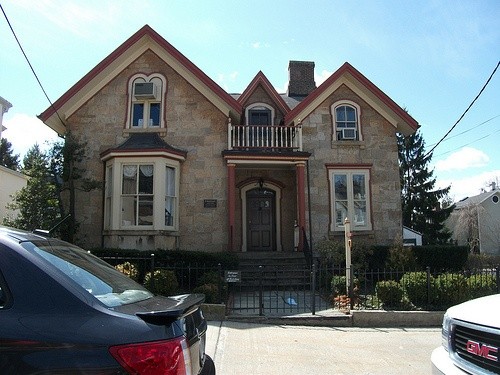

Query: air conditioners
[341,128,355,140]
[134,82,157,99]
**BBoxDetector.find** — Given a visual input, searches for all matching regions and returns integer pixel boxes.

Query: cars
[429,293,500,374]
[0,225,217,375]
